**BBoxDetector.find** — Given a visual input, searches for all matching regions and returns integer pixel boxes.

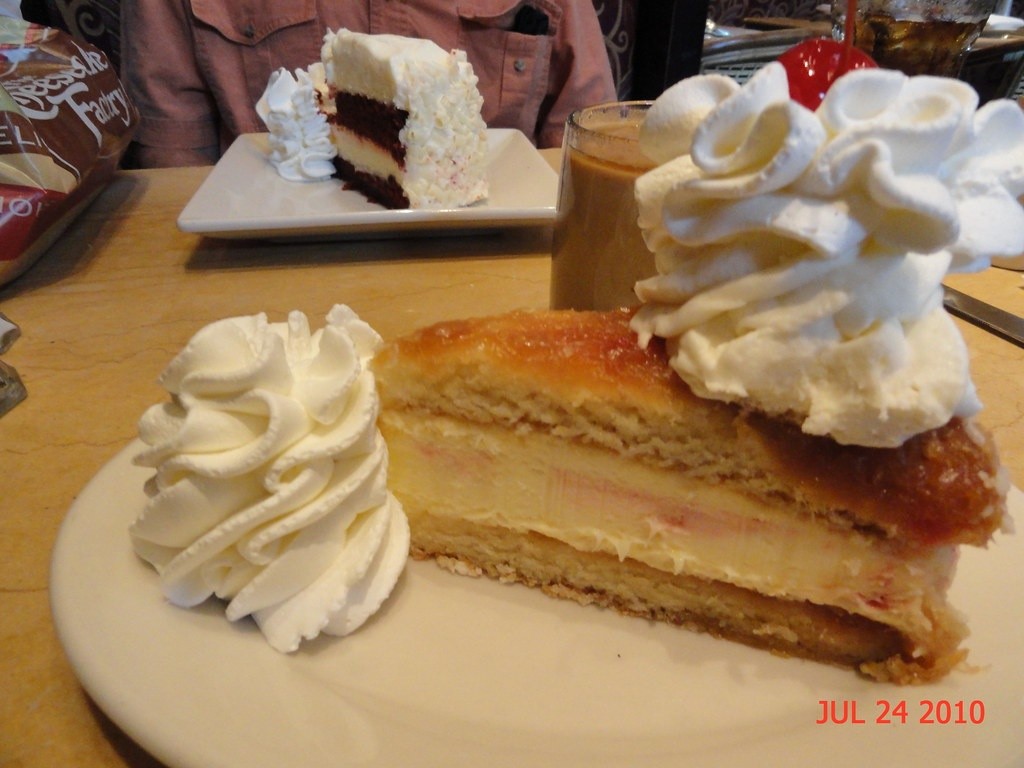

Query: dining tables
[0,15,1024,768]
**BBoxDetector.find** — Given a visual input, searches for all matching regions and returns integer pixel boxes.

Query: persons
[116,0,619,169]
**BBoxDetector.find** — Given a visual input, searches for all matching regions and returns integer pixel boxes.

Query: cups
[550,100,668,313]
[828,0,997,80]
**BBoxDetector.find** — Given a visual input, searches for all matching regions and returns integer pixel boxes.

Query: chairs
[699,26,831,86]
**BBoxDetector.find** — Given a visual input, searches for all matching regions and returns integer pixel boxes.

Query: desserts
[255,28,489,211]
[124,0,1024,687]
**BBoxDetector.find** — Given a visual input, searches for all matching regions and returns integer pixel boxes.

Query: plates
[176,128,563,240]
[49,433,1024,768]
